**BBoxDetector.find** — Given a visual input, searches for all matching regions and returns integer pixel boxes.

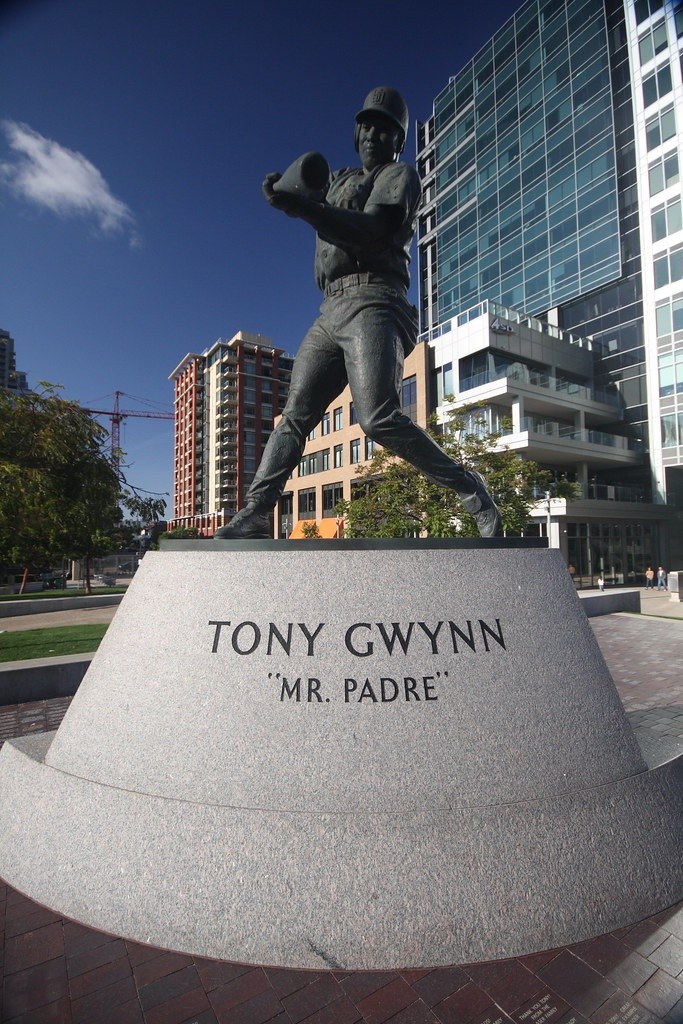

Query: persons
[568,564,576,582]
[213,86,504,540]
[656,566,668,591]
[645,567,654,590]
[598,576,604,592]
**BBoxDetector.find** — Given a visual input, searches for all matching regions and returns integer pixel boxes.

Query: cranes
[67,388,175,510]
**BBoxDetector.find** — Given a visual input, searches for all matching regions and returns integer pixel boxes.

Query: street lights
[282,518,292,539]
[335,514,346,539]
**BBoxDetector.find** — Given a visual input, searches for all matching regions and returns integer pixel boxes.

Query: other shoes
[645,588,648,590]
[651,589,653,590]
[656,589,660,591]
[664,589,666,591]
